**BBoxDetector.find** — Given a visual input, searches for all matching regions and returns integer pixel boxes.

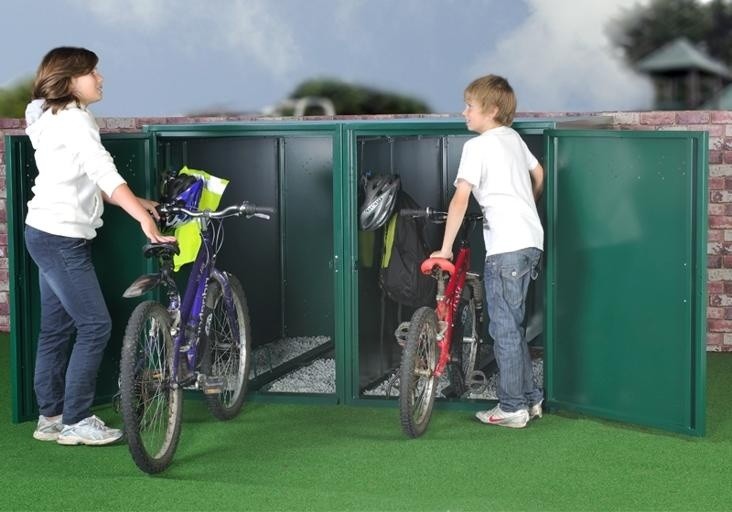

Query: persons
[23,44,176,446]
[430,74,545,428]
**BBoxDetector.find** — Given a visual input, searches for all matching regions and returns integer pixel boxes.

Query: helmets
[357,168,403,235]
[154,169,204,236]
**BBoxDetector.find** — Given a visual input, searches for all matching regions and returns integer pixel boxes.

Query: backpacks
[377,187,440,308]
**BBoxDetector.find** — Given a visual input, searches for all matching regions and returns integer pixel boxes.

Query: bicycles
[111,198,278,477]
[393,204,488,442]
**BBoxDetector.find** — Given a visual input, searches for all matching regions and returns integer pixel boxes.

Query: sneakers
[30,413,63,443]
[528,396,547,423]
[55,413,127,449]
[474,402,530,428]
